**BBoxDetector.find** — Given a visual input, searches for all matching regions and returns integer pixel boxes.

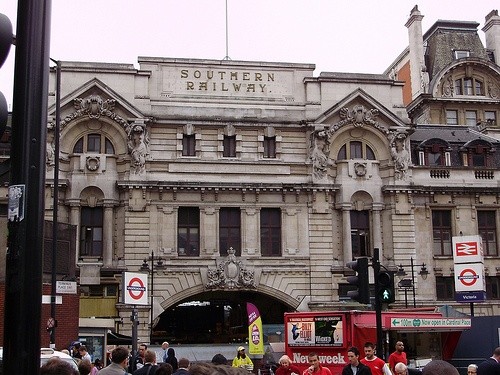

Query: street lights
[140,249,166,345]
[397,256,430,308]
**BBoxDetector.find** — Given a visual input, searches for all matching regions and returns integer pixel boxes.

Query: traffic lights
[347,257,371,305]
[377,271,395,305]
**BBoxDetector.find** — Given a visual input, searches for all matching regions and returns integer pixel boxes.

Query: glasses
[466,371,477,374]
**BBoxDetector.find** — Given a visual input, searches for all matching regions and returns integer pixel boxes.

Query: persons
[40,342,500,375]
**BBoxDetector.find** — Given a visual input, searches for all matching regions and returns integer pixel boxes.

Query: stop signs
[454,263,484,292]
[124,272,148,306]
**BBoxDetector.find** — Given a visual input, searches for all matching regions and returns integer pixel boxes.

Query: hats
[238,346,245,351]
